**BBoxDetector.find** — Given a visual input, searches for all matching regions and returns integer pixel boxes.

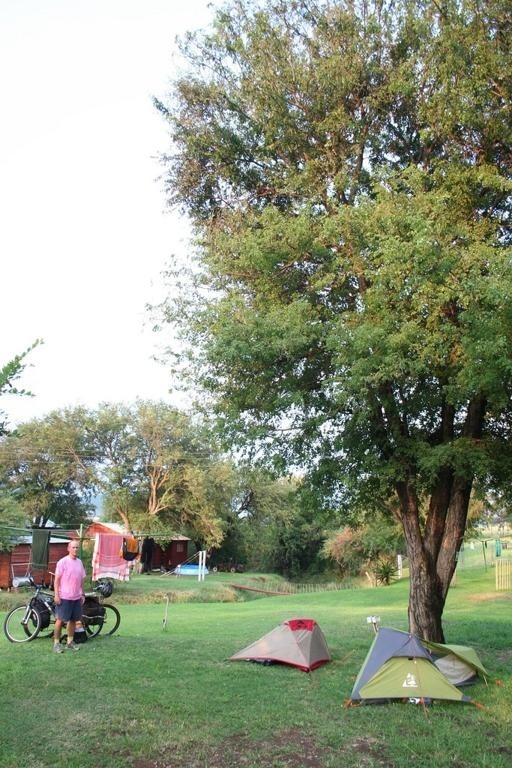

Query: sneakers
[64,640,80,652]
[52,643,65,654]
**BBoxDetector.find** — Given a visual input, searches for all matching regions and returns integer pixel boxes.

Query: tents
[346,628,490,710]
[231,618,331,674]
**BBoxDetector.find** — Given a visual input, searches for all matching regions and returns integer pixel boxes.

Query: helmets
[101,581,113,598]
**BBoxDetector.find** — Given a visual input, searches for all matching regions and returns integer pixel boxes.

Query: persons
[52,542,87,653]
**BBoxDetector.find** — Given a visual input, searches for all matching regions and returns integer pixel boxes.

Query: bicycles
[4,578,120,643]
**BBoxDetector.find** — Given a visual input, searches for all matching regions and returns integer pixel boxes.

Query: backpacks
[81,597,106,626]
[31,601,50,630]
